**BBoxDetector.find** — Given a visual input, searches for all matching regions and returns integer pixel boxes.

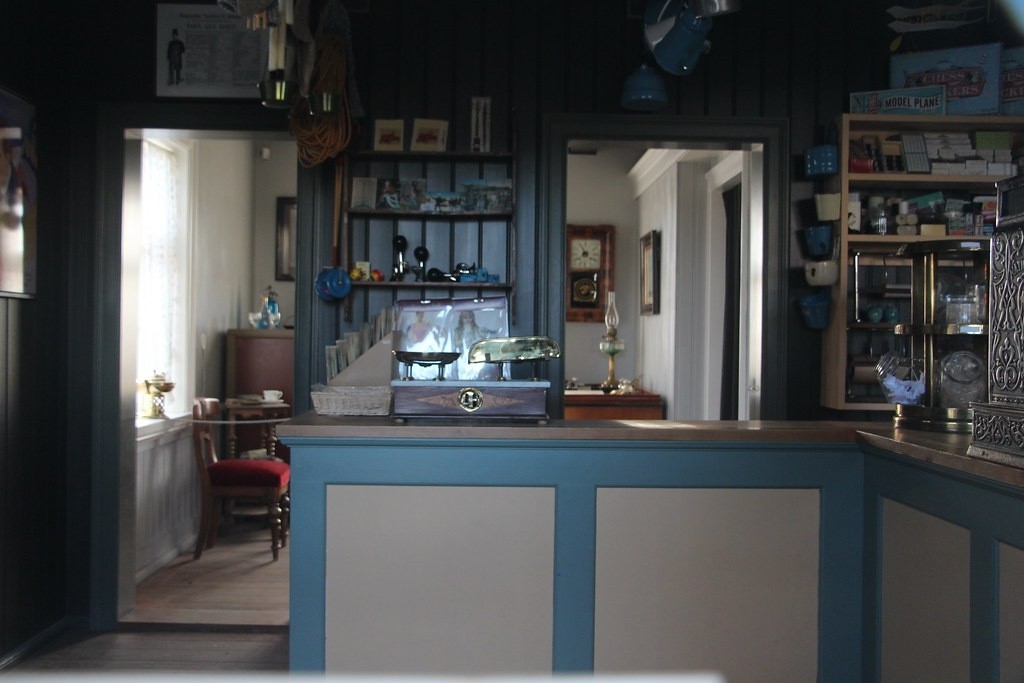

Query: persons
[168,29,185,85]
[455,310,484,346]
[409,312,430,341]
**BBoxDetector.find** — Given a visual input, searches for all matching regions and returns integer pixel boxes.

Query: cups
[263,389,283,400]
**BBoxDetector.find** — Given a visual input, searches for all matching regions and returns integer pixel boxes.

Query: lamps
[620,1,743,112]
[600,291,625,392]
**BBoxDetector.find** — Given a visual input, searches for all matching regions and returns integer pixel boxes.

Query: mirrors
[276,196,300,282]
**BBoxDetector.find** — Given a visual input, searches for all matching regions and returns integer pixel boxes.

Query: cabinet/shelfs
[822,110,1023,411]
[339,147,518,325]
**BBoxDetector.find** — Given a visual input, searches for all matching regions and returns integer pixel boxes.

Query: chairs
[195,397,290,561]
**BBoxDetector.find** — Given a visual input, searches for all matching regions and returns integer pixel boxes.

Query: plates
[261,399,284,404]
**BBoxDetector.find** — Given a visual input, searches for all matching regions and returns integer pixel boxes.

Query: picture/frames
[0,86,42,299]
[640,227,661,315]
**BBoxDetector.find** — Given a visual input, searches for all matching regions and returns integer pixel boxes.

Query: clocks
[565,223,613,323]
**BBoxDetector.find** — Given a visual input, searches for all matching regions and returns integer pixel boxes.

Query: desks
[225,326,295,464]
[564,388,663,420]
[224,400,290,459]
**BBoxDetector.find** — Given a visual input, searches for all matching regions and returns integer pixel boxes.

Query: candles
[269,12,285,70]
